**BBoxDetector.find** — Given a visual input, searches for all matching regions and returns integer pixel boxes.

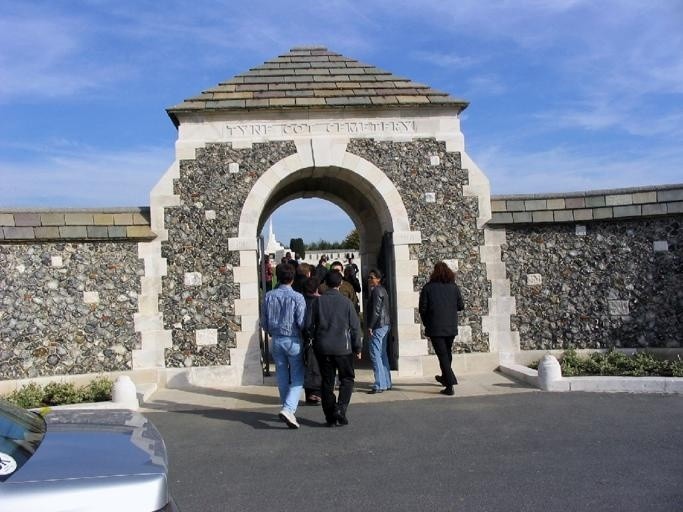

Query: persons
[258,251,391,428]
[418,262,463,395]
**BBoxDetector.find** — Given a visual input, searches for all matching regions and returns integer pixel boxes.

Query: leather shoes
[325,410,348,427]
[435,375,458,395]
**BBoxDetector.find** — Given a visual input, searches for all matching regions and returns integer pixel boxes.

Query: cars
[0,397,176,511]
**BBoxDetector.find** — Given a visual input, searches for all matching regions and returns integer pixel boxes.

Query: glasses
[368,276,376,279]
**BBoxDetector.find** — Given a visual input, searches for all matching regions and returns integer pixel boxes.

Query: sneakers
[306,394,321,404]
[368,388,391,394]
[278,409,300,429]
